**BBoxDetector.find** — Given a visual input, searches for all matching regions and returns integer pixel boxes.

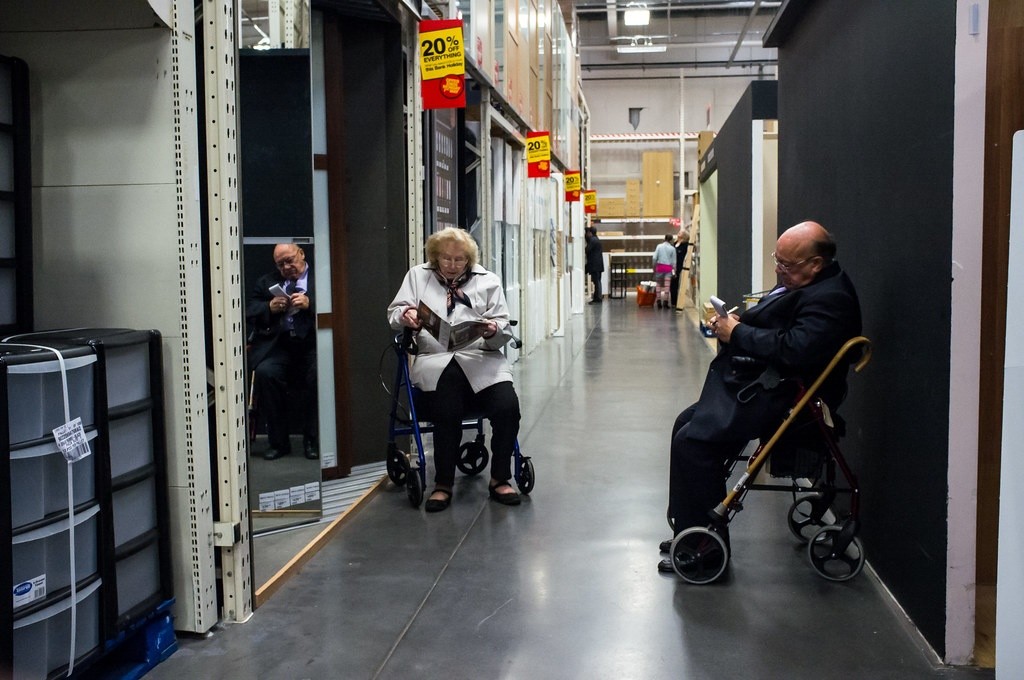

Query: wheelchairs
[667,335,877,586]
[379,319,536,509]
[245,325,320,455]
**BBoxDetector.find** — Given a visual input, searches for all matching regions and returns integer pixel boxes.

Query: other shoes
[589,299,602,305]
[424,489,453,513]
[488,478,521,506]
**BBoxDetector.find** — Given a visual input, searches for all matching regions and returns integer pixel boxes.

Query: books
[416,299,491,352]
[268,284,291,302]
[710,296,729,317]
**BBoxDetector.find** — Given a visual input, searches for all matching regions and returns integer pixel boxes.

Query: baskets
[636,284,657,307]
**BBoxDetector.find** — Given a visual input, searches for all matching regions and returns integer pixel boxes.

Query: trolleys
[639,271,657,308]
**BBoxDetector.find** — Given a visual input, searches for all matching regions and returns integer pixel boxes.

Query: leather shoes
[303,438,318,459]
[660,535,698,552]
[265,443,292,460]
[658,547,705,573]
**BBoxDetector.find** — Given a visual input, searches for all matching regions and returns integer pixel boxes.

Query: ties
[282,277,300,323]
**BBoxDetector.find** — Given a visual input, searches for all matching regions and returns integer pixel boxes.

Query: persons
[387,227,523,512]
[653,234,677,308]
[248,244,319,459]
[658,221,863,573]
[670,231,690,308]
[585,226,604,304]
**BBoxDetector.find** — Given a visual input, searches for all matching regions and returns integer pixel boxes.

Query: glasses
[437,256,469,268]
[276,249,301,268]
[772,250,816,270]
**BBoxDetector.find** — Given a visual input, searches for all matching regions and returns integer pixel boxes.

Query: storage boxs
[642,150,674,217]
[597,197,624,217]
[699,303,718,337]
[626,178,640,217]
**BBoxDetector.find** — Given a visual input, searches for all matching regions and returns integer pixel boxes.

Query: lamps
[617,36,668,54]
[624,1,649,26]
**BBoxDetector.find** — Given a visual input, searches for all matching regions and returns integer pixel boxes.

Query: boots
[664,300,670,309]
[657,300,662,310]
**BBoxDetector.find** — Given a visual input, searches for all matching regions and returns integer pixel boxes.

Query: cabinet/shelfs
[0,328,176,680]
[595,217,678,291]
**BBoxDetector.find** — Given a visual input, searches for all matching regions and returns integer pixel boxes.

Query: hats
[585,227,597,235]
